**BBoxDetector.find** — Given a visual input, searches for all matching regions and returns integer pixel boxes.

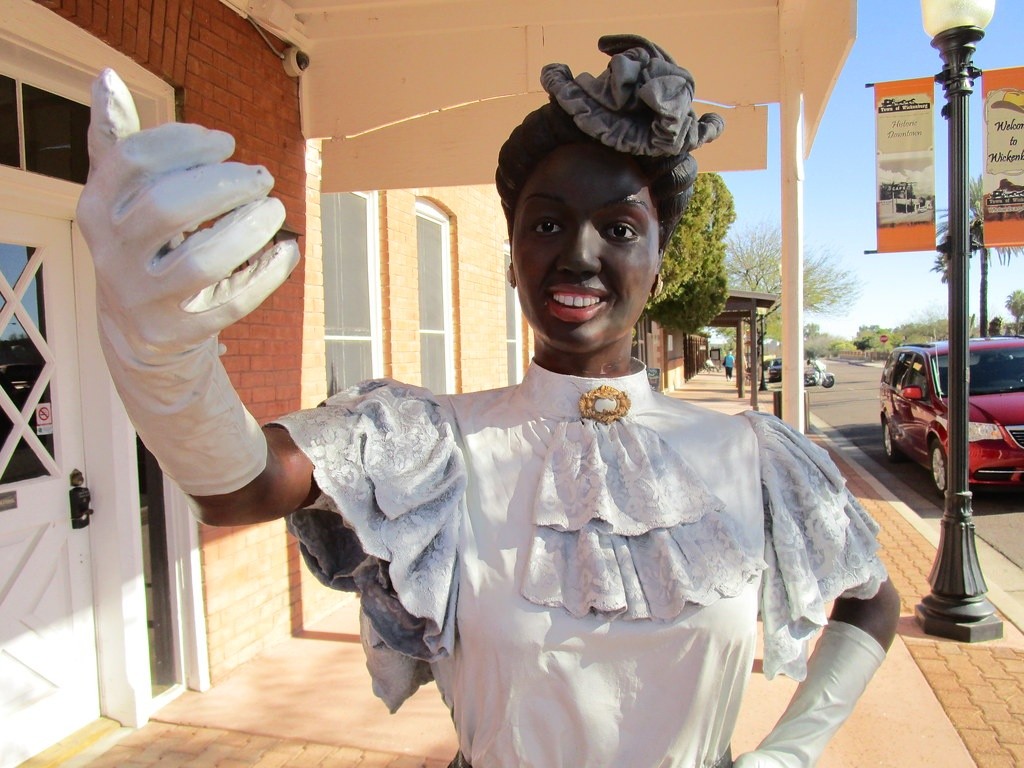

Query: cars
[768,358,783,382]
[0,363,44,410]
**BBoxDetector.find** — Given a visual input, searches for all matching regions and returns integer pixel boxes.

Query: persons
[971,351,1023,390]
[72,32,899,768]
[724,351,736,382]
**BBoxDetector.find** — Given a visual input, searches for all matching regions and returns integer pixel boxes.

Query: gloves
[731,617,893,768]
[78,64,302,500]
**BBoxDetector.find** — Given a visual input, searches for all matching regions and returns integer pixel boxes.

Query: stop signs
[881,335,887,341]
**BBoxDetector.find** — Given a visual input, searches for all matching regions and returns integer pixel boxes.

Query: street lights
[918,0,1003,644]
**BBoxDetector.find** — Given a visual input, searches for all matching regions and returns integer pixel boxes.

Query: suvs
[879,334,1024,502]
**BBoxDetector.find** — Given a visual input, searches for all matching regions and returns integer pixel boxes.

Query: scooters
[805,355,836,389]
